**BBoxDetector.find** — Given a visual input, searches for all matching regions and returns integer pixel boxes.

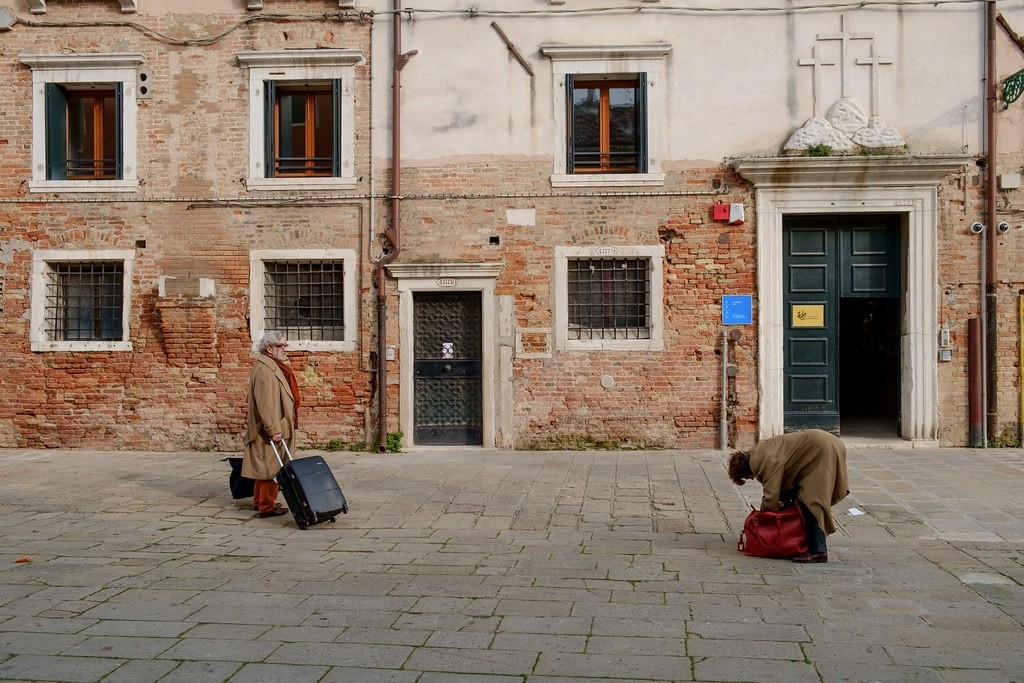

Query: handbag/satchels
[737,499,808,558]
[220,458,255,499]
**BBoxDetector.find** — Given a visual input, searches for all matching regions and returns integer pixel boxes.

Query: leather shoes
[254,501,282,511]
[791,550,828,563]
[260,507,288,518]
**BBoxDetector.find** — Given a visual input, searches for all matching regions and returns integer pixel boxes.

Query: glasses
[274,343,288,348]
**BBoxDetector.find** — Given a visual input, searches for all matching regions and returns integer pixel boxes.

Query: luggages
[269,434,349,530]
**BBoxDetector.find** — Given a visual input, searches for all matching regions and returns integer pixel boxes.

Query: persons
[728,429,850,562]
[242,332,300,518]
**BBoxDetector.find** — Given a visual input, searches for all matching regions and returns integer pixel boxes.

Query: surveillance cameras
[970,222,983,234]
[997,222,1009,233]
